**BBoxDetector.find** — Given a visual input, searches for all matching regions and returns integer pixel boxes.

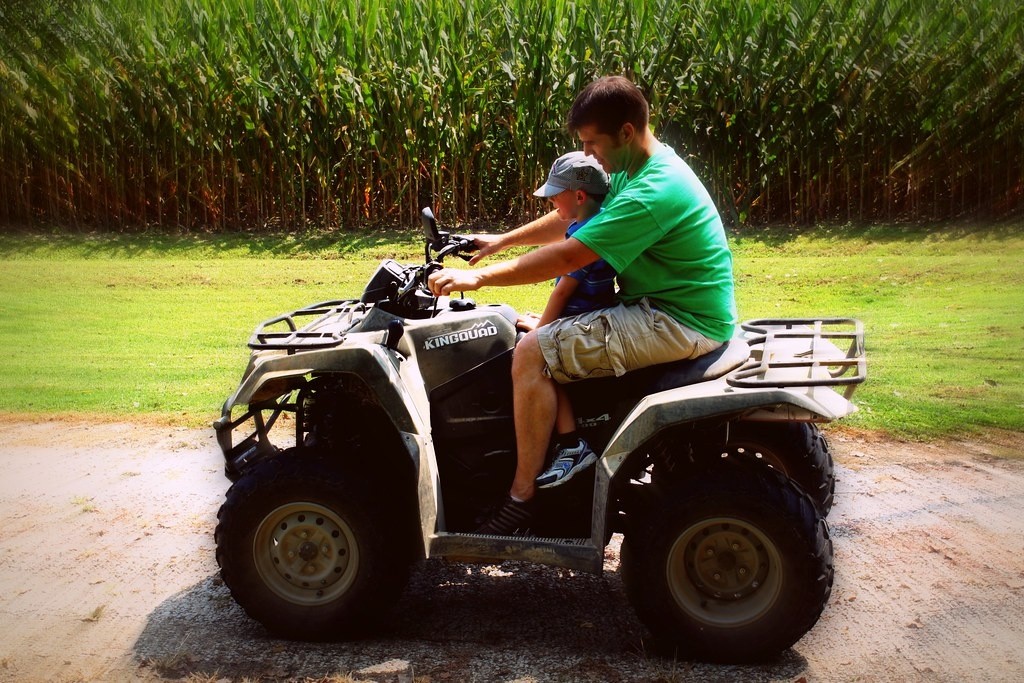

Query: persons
[428,76,737,536]
[517,150,621,488]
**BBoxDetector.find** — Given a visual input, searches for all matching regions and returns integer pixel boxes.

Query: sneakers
[477,494,549,534]
[534,439,600,489]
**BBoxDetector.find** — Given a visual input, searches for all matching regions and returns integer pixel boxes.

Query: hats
[532,151,609,199]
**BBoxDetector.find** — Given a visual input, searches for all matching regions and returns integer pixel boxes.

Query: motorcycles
[213,206,867,664]
